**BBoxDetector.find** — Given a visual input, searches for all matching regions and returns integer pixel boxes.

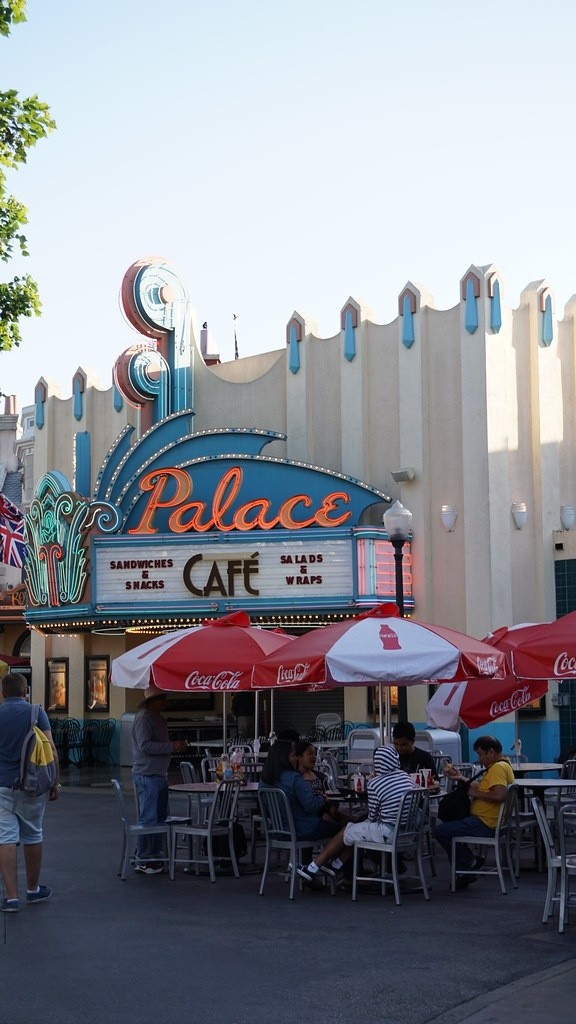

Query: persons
[0,673,61,912]
[289,746,419,881]
[260,741,353,890]
[431,734,515,890]
[132,686,187,874]
[273,727,352,822]
[393,721,441,784]
[230,693,255,738]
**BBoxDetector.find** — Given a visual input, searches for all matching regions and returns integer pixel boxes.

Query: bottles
[216,761,243,783]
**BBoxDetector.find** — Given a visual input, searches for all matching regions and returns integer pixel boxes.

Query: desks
[188,738,254,781]
[325,784,448,894]
[167,780,260,875]
[310,739,348,775]
[509,762,567,841]
[508,779,576,872]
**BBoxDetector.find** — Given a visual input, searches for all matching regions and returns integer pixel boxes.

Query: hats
[136,686,169,708]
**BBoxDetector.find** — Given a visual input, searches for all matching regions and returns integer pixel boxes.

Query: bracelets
[54,784,61,788]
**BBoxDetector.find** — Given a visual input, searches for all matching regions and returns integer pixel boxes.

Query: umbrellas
[108,610,303,761]
[504,610,576,680]
[251,601,506,746]
[425,622,564,733]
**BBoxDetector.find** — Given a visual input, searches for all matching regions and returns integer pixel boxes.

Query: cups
[410,773,422,787]
[354,776,364,792]
[419,769,432,788]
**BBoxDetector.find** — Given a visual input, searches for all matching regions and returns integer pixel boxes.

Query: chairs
[49,713,576,933]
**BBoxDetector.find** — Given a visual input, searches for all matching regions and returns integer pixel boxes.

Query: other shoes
[469,855,484,871]
[450,874,476,889]
[383,862,411,891]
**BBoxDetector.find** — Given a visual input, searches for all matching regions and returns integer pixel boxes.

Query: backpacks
[204,815,247,868]
[20,704,56,796]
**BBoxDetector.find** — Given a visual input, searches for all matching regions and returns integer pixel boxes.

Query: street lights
[383,500,412,722]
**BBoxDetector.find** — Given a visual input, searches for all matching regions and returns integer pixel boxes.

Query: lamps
[560,506,576,531]
[441,504,458,532]
[512,502,528,529]
[17,458,28,481]
[391,468,416,482]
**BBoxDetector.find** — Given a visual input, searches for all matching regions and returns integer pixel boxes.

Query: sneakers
[135,862,164,874]
[320,861,339,877]
[27,885,53,903]
[0,898,20,912]
[289,862,315,881]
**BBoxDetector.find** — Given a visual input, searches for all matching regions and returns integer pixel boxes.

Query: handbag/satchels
[437,782,472,822]
